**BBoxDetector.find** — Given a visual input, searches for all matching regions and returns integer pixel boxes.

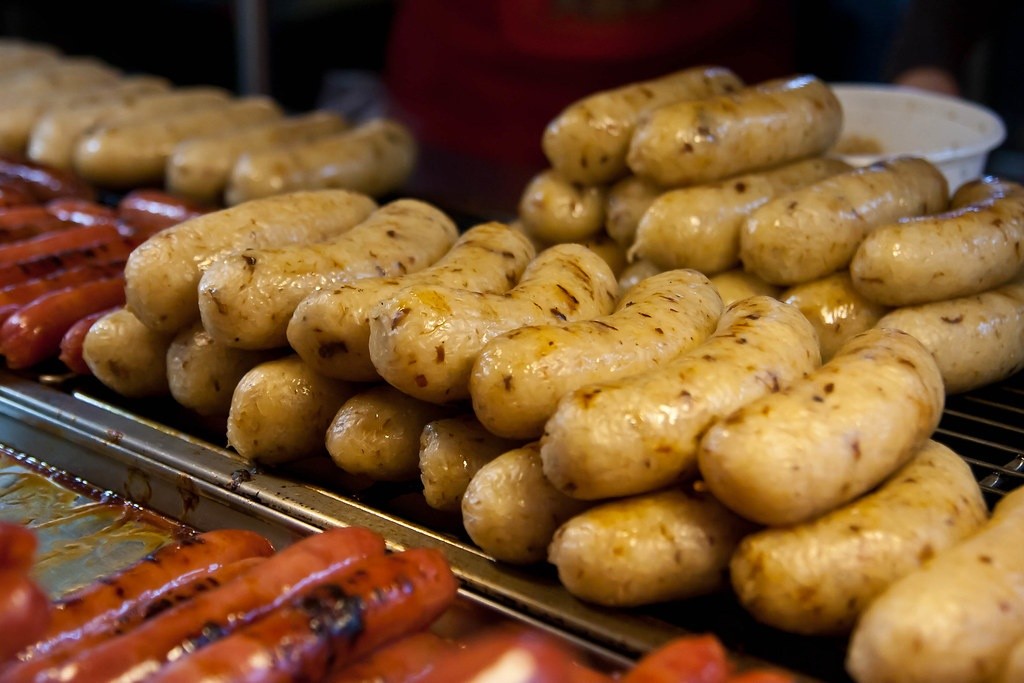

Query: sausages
[0,36,1024,683]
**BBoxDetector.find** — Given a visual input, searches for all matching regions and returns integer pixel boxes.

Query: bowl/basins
[827,80,1008,197]
